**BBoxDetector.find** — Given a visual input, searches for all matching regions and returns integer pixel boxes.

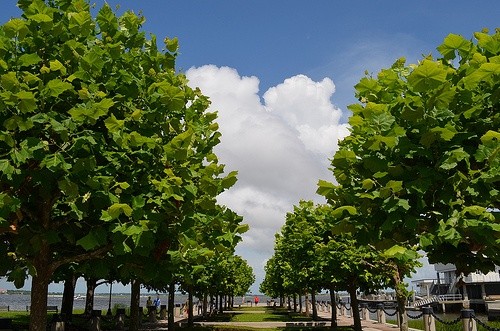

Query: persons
[196,295,204,315]
[146,297,152,313]
[255,296,259,307]
[208,299,215,313]
[185,302,189,317]
[153,296,160,306]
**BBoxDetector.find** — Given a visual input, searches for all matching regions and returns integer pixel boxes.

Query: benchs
[47,306,58,312]
